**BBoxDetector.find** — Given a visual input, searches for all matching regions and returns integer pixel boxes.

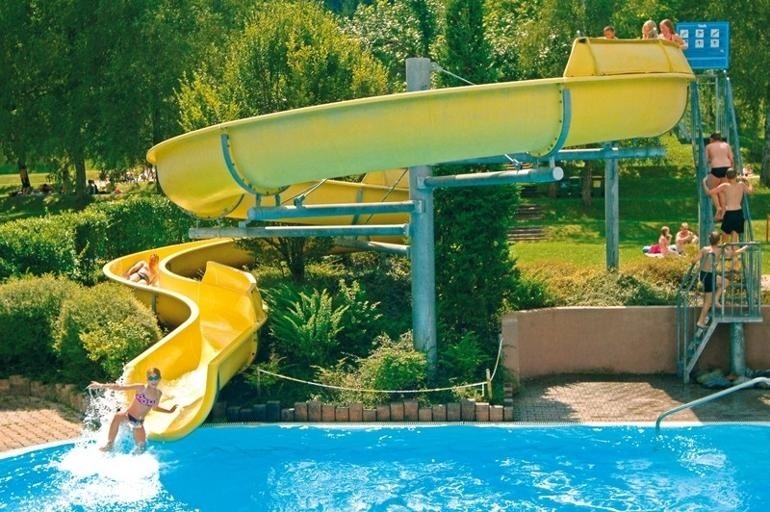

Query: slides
[102,70,694,441]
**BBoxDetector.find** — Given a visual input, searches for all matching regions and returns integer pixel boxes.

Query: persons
[656,133,757,331]
[600,24,620,41]
[656,19,689,51]
[639,20,659,39]
[125,254,162,288]
[18,164,159,196]
[87,368,180,455]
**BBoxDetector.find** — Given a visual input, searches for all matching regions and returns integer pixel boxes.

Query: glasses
[148,375,160,381]
